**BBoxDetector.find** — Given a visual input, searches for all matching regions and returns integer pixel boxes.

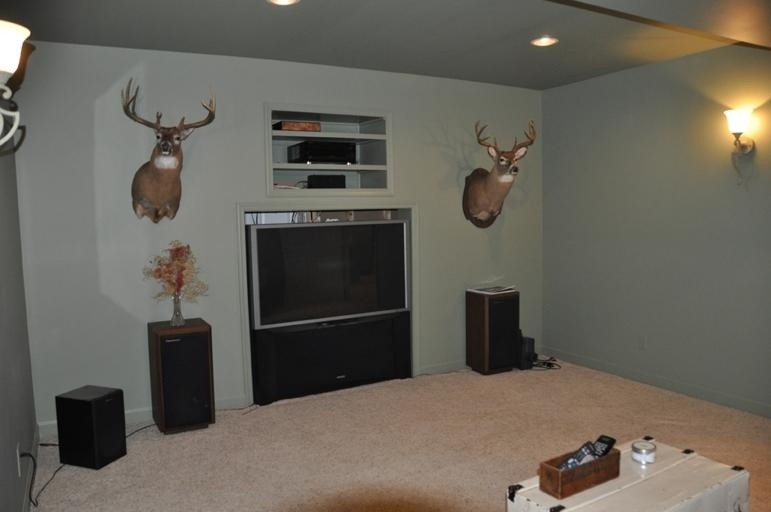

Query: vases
[168,296,187,328]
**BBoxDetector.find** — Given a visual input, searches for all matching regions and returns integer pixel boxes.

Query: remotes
[593,434,616,458]
[556,441,596,471]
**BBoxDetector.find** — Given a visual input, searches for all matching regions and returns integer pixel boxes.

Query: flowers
[140,239,209,322]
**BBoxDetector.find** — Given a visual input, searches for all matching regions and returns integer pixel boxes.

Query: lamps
[0,20,31,146]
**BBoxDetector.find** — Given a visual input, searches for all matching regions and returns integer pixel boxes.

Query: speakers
[465,286,520,375]
[147,317,216,435]
[54,385,127,470]
[517,336,538,370]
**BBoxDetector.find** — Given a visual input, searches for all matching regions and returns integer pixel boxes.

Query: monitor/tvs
[246,218,412,331]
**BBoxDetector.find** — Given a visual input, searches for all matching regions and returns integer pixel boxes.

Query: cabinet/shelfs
[262,101,395,198]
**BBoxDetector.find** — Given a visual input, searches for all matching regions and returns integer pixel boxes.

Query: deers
[459,117,538,229]
[118,77,218,225]
